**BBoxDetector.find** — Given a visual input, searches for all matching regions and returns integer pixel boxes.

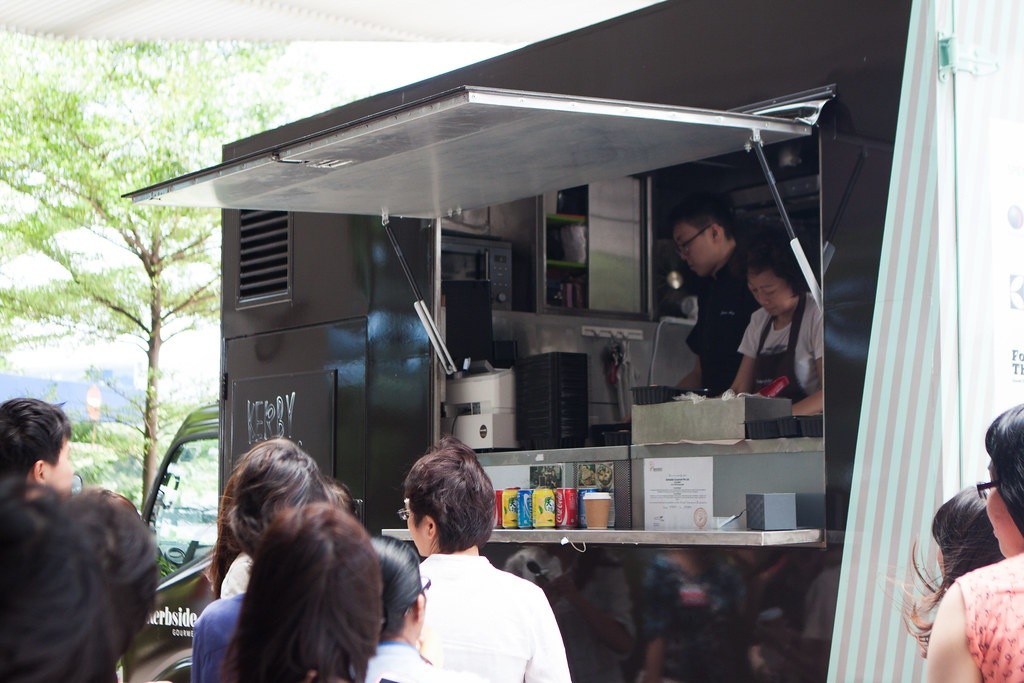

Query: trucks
[121,0,1024,683]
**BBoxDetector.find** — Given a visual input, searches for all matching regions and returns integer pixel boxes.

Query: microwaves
[440,236,513,311]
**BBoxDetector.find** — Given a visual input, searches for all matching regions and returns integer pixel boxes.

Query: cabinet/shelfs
[489,174,653,321]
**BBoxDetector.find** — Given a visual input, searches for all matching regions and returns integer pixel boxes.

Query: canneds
[493,487,599,530]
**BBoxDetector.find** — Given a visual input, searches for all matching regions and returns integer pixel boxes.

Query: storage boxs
[514,351,824,449]
[745,492,798,531]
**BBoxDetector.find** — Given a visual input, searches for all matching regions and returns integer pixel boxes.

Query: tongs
[755,376,790,398]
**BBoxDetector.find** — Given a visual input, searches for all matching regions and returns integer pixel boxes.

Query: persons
[0,397,157,682]
[901,403,1024,683]
[668,194,769,397]
[503,543,842,683]
[397,433,573,683]
[188,437,487,683]
[730,243,824,417]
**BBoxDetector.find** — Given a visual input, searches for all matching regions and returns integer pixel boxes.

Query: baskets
[744,413,823,439]
[631,385,711,404]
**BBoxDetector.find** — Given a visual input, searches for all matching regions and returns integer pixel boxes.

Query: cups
[583,491,611,531]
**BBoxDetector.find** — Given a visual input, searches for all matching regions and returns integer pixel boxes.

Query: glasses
[975,478,999,500]
[674,221,714,255]
[404,574,432,618]
[397,507,411,522]
